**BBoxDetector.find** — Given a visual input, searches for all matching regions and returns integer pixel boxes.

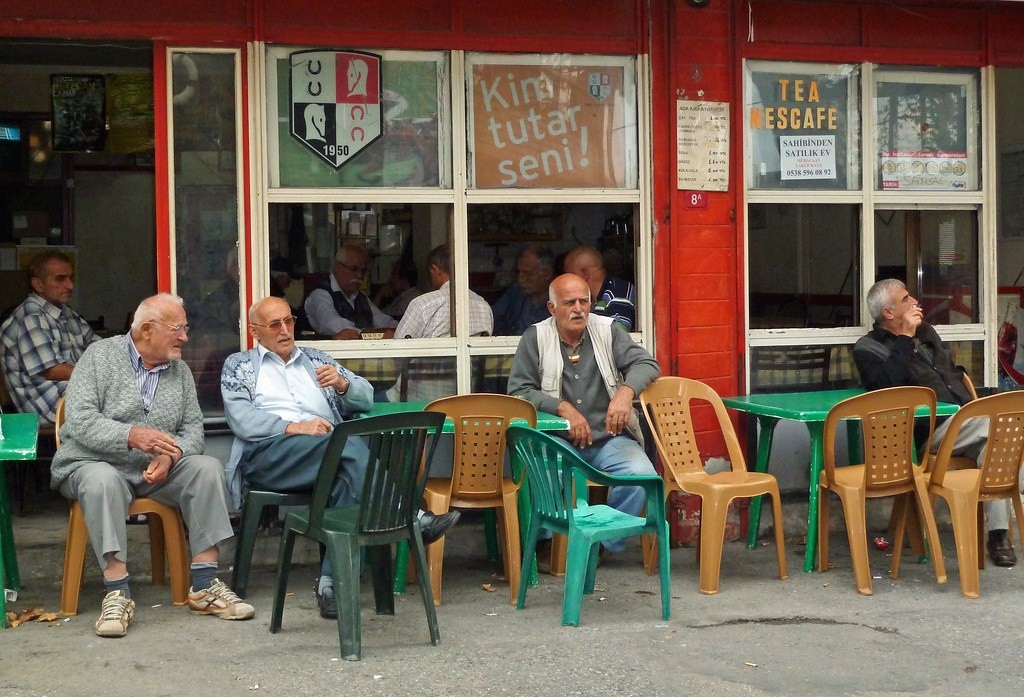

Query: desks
[0,413,40,627]
[721,389,961,574]
[352,402,571,596]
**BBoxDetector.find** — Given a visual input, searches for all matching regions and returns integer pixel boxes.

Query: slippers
[125,513,148,524]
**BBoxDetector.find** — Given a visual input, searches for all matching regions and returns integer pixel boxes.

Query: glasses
[339,261,367,274]
[148,318,189,334]
[253,316,297,332]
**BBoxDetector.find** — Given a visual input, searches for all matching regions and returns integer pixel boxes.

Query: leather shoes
[986,533,1017,568]
[315,583,338,617]
[410,510,461,548]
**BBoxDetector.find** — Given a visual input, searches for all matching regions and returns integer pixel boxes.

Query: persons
[509,274,661,572]
[386,257,426,320]
[0,249,151,523]
[564,244,636,331]
[300,244,399,332]
[854,278,1024,568]
[368,244,494,401]
[483,245,556,334]
[220,296,461,619]
[271,275,360,340]
[52,292,256,637]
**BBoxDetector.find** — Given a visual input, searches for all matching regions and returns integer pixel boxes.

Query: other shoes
[534,538,552,572]
[597,542,605,569]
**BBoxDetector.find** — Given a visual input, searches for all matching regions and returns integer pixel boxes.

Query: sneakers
[189,578,256,620]
[95,590,135,636]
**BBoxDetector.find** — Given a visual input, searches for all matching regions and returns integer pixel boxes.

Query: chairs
[749,339,833,394]
[888,373,1013,568]
[819,385,947,595]
[402,393,538,604]
[271,411,448,661]
[56,392,191,616]
[401,330,489,404]
[231,485,330,599]
[890,390,1024,598]
[551,472,653,578]
[504,426,671,626]
[639,376,789,594]
